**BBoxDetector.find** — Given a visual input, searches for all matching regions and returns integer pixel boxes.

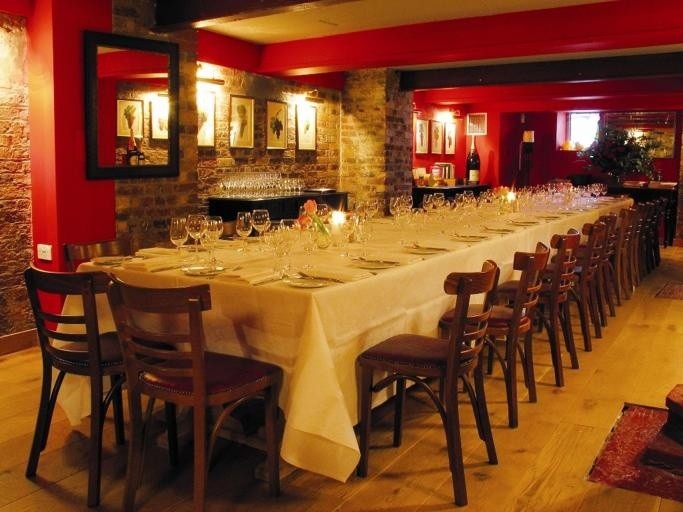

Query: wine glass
[237,212,252,255]
[343,182,604,258]
[265,219,299,272]
[216,170,311,201]
[170,215,224,265]
[300,222,319,272]
[251,210,268,245]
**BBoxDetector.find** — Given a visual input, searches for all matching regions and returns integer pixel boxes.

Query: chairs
[538,223,607,351]
[355,259,499,506]
[105,272,284,511]
[62,239,131,272]
[598,198,668,315]
[438,242,550,427]
[23,266,179,508]
[488,228,581,387]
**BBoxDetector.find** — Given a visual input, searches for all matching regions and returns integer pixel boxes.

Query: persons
[232,104,251,146]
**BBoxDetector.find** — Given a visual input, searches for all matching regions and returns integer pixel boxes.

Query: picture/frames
[599,111,679,161]
[466,113,488,136]
[295,103,317,153]
[445,121,455,155]
[196,91,215,151]
[116,99,145,139]
[149,93,169,144]
[228,94,255,150]
[415,118,429,155]
[430,120,444,155]
[265,99,288,150]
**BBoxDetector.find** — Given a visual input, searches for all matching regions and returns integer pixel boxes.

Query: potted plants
[576,124,663,186]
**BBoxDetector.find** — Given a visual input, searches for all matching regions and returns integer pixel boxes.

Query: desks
[594,175,677,248]
[206,190,348,226]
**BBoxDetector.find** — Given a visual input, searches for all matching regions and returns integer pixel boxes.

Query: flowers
[123,105,136,129]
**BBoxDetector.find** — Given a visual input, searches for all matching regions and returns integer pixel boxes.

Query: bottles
[139,139,146,164]
[129,132,139,165]
[465,135,484,185]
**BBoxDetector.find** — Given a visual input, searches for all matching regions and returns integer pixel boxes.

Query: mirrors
[81,28,179,180]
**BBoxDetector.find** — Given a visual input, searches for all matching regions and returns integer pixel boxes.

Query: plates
[454,232,489,243]
[544,209,578,222]
[359,261,394,269]
[512,215,537,226]
[184,265,223,277]
[93,255,128,265]
[291,278,328,288]
[408,243,448,258]
[482,223,513,236]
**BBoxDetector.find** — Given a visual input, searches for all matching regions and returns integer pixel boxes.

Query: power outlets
[37,244,53,261]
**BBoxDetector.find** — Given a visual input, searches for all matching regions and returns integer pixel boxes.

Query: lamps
[518,130,535,169]
[412,184,489,208]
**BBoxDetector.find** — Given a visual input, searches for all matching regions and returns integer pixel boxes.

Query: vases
[128,129,135,151]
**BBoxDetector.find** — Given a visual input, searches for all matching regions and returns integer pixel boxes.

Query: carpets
[586,402,682,502]
[654,283,683,300]
